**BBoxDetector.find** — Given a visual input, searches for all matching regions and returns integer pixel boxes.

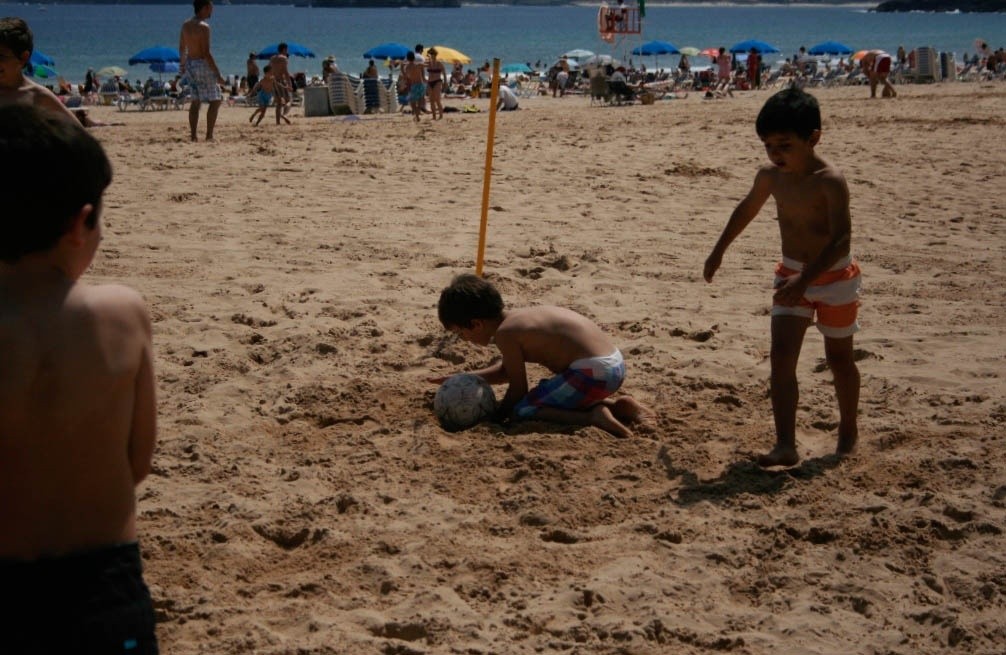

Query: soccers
[433,372,497,430]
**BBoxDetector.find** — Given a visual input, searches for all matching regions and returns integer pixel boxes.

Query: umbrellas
[586,54,617,66]
[96,66,128,77]
[559,49,597,71]
[22,48,58,79]
[255,42,319,60]
[729,40,780,53]
[128,46,182,94]
[808,41,855,55]
[679,46,701,56]
[701,48,727,56]
[631,40,680,70]
[500,64,532,72]
[363,42,472,64]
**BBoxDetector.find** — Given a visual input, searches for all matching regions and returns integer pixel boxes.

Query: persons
[605,0,627,31]
[437,274,656,438]
[524,43,1006,106]
[249,44,297,126]
[400,44,448,122]
[0,114,155,655]
[46,53,260,106]
[308,56,524,111]
[75,109,127,127]
[703,87,861,466]
[179,0,226,142]
[0,17,87,128]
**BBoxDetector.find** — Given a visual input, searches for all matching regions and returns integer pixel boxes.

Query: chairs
[98,92,186,111]
[228,83,257,107]
[589,75,642,106]
